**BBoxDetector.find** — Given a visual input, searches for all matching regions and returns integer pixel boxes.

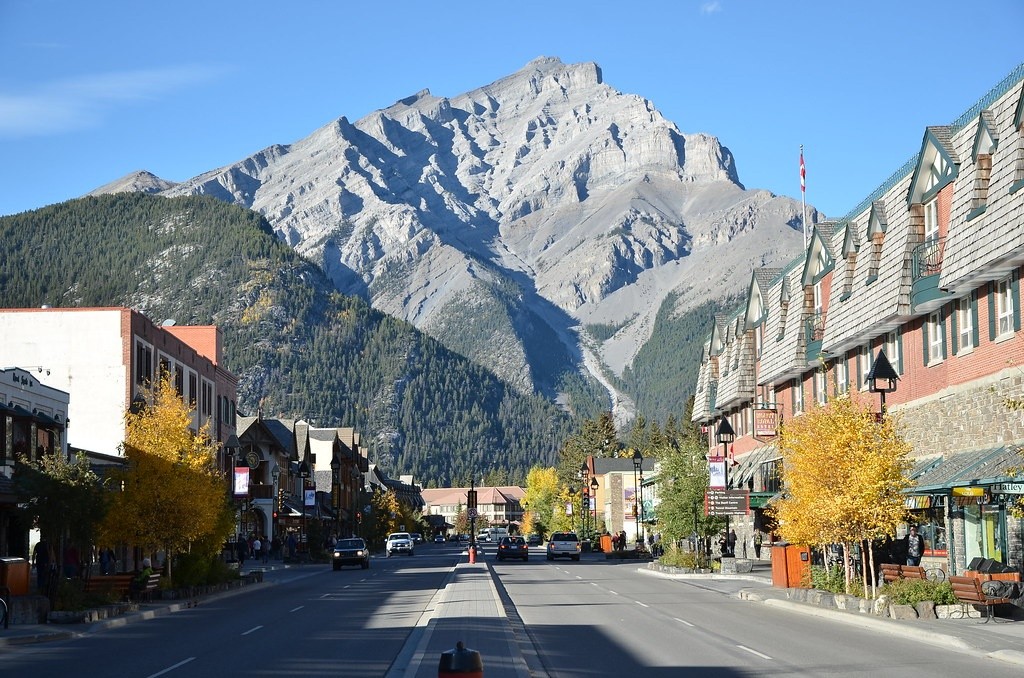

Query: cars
[449,533,470,542]
[434,534,445,544]
[496,536,529,562]
[410,533,423,545]
[529,534,543,545]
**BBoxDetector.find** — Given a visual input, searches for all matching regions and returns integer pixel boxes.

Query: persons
[718,532,727,554]
[612,530,626,551]
[31,535,56,593]
[124,558,153,602]
[729,529,737,553]
[235,532,298,564]
[752,529,762,560]
[649,532,664,557]
[900,524,925,566]
[98,546,115,576]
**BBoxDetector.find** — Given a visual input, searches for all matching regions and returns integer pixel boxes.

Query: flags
[799,157,806,191]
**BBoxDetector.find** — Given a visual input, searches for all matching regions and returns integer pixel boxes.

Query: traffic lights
[581,486,590,511]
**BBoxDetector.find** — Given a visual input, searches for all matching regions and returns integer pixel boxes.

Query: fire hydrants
[468,546,476,564]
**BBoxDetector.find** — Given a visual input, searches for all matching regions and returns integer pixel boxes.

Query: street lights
[864,348,902,565]
[567,487,575,533]
[632,447,645,552]
[579,461,590,541]
[591,477,599,530]
[714,415,738,557]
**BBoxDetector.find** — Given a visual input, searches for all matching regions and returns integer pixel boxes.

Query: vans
[475,527,508,544]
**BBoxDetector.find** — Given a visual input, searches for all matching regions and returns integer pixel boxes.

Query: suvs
[545,530,581,562]
[331,537,370,572]
[384,532,415,558]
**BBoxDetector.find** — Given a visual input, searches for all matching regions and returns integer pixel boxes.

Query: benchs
[946,576,1008,624]
[881,564,945,588]
[83,575,133,603]
[136,574,160,603]
